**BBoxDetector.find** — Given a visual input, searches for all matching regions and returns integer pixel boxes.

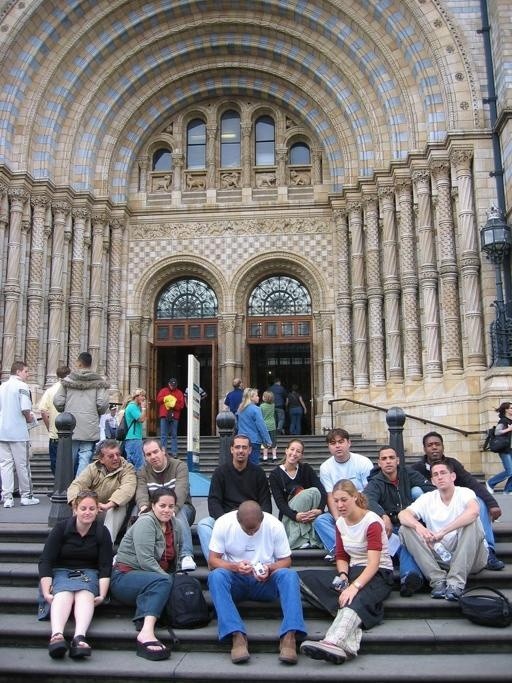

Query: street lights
[478,204,511,366]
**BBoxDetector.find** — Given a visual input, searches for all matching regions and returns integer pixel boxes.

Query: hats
[168,377,177,386]
[109,403,117,411]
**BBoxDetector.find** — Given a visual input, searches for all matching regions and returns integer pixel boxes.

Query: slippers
[69,633,92,658]
[135,638,171,661]
[48,631,68,659]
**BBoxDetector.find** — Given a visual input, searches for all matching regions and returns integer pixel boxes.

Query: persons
[39,428,503,665]
[224,376,306,464]
[485,402,512,493]
[1,361,41,508]
[39,353,207,497]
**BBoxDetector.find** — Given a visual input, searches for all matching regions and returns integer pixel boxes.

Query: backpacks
[158,568,211,646]
[458,585,512,629]
[116,401,136,441]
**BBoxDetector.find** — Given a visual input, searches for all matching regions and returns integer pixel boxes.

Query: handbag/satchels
[488,434,511,453]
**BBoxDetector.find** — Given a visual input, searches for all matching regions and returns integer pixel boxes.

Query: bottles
[331,576,349,590]
[434,539,452,564]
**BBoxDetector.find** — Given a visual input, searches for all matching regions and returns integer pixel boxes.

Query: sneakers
[229,632,250,663]
[3,497,15,508]
[488,554,505,571]
[430,582,445,600]
[20,495,40,506]
[486,481,494,494]
[259,458,278,464]
[442,584,461,602]
[299,640,345,666]
[278,631,300,665]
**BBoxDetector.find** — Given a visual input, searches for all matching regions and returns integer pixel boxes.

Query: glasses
[76,491,98,497]
